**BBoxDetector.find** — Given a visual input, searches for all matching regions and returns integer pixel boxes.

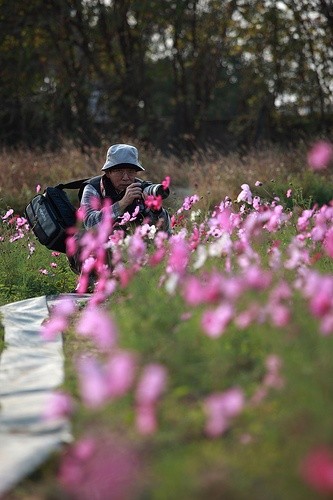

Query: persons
[68,144,169,277]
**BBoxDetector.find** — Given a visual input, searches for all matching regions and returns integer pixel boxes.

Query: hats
[100,144,145,173]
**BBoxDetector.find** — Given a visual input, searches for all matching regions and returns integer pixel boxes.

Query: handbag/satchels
[25,177,101,254]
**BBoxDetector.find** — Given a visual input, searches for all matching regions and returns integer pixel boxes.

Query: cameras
[135,180,169,203]
